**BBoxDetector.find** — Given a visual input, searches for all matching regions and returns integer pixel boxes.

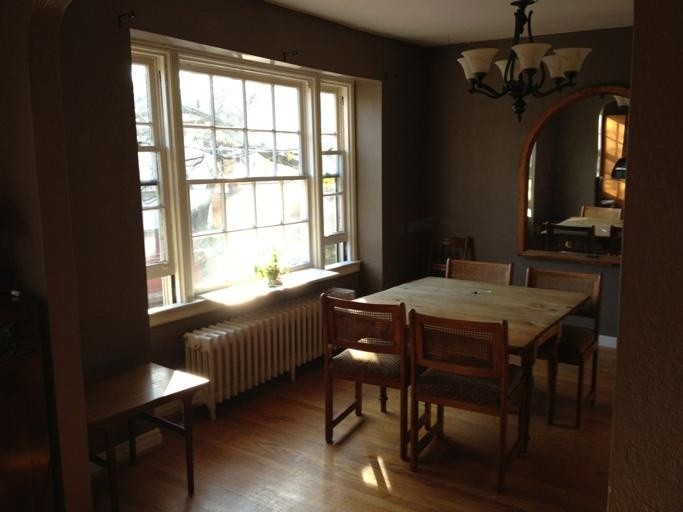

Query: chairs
[545,219,595,250]
[579,204,622,222]
[317,292,432,461]
[444,257,513,284]
[524,265,605,429]
[406,307,529,493]
[426,233,472,276]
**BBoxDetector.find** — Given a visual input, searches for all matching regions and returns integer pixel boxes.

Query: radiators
[179,286,358,421]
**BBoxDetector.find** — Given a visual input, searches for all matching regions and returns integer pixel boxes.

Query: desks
[85,360,213,511]
[344,275,592,453]
[540,214,621,254]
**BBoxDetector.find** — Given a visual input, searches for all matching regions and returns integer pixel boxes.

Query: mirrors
[517,93,632,262]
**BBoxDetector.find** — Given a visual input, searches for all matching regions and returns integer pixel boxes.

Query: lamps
[455,1,593,121]
